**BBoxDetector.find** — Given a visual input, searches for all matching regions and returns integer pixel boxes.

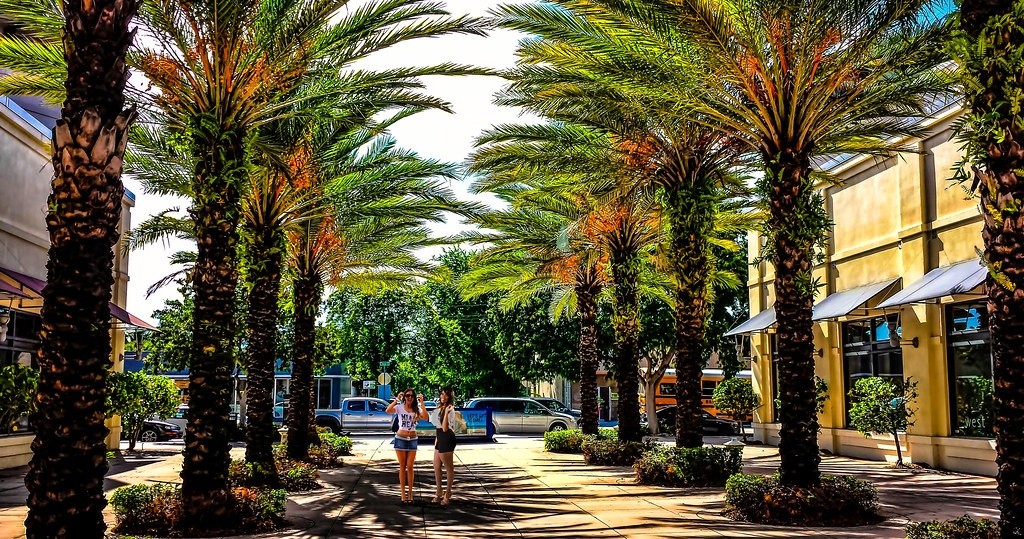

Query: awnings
[812,277,901,321]
[722,307,776,339]
[872,257,989,310]
[0,279,28,298]
[1,268,160,332]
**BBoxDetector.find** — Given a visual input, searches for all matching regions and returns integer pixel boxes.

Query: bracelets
[396,398,400,402]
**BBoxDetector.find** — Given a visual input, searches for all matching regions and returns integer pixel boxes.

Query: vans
[149,404,188,438]
[466,398,578,437]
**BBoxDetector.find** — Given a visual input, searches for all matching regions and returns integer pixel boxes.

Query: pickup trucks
[315,395,399,434]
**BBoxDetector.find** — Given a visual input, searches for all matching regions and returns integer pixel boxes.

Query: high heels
[431,492,444,502]
[408,496,414,504]
[441,492,451,505]
[400,496,407,504]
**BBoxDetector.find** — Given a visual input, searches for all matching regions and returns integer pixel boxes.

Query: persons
[428,387,456,505]
[385,389,429,504]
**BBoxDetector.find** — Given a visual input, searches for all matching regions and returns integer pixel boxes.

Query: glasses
[405,394,415,397]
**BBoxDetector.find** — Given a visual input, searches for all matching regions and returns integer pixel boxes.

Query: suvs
[530,397,583,425]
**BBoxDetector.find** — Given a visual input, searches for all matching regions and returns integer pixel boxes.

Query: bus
[639,367,752,425]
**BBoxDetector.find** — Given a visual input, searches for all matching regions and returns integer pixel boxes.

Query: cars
[423,401,437,411]
[121,417,184,442]
[641,406,738,436]
